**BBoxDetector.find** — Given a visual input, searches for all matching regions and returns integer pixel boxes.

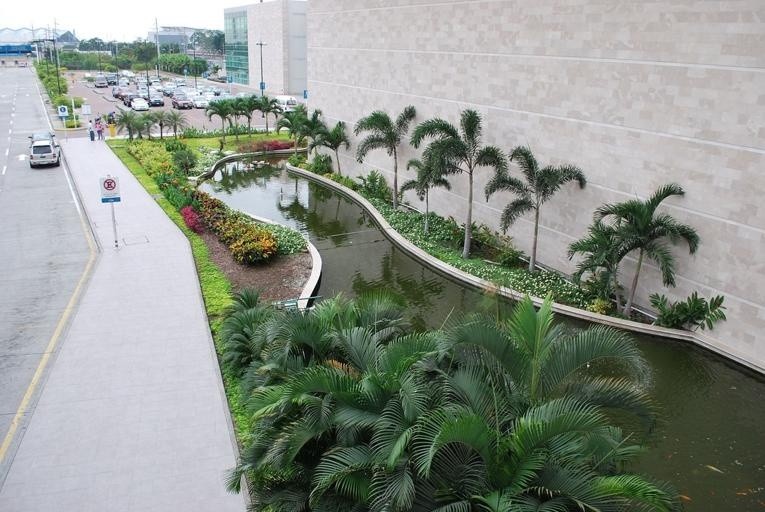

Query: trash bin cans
[109,123,116,138]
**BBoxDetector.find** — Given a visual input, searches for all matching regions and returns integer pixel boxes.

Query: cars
[29,140,61,168]
[163,79,249,109]
[28,132,55,141]
[94,68,163,110]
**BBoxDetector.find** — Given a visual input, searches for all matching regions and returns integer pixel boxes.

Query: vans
[273,95,297,113]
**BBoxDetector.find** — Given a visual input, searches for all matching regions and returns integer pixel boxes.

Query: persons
[108,112,115,124]
[95,119,103,142]
[101,127,105,137]
[88,119,96,141]
